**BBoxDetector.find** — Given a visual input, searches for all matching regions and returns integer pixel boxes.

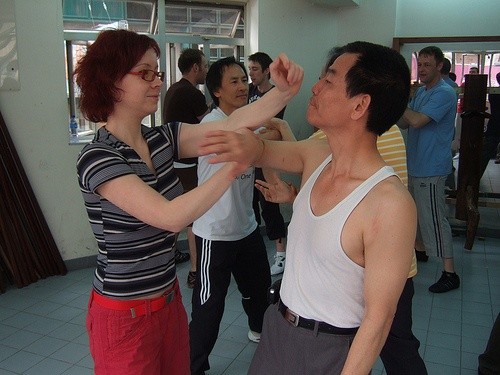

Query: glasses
[125,69,165,82]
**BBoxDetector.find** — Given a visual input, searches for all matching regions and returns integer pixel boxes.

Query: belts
[279,296,360,336]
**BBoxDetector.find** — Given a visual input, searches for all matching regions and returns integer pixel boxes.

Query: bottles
[69,115,79,143]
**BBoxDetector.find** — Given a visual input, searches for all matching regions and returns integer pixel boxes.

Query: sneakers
[174,249,189,264]
[187,271,196,288]
[414,248,428,262]
[270,253,285,275]
[247,328,261,343]
[429,271,460,293]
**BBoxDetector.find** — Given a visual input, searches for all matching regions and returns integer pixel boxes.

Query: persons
[396,46,460,294]
[441,58,500,164]
[70,28,306,375]
[186,56,281,375]
[478,312,500,375]
[161,47,288,289]
[258,44,430,375]
[196,40,418,375]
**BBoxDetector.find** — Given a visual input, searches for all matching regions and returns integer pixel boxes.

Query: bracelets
[252,139,266,163]
